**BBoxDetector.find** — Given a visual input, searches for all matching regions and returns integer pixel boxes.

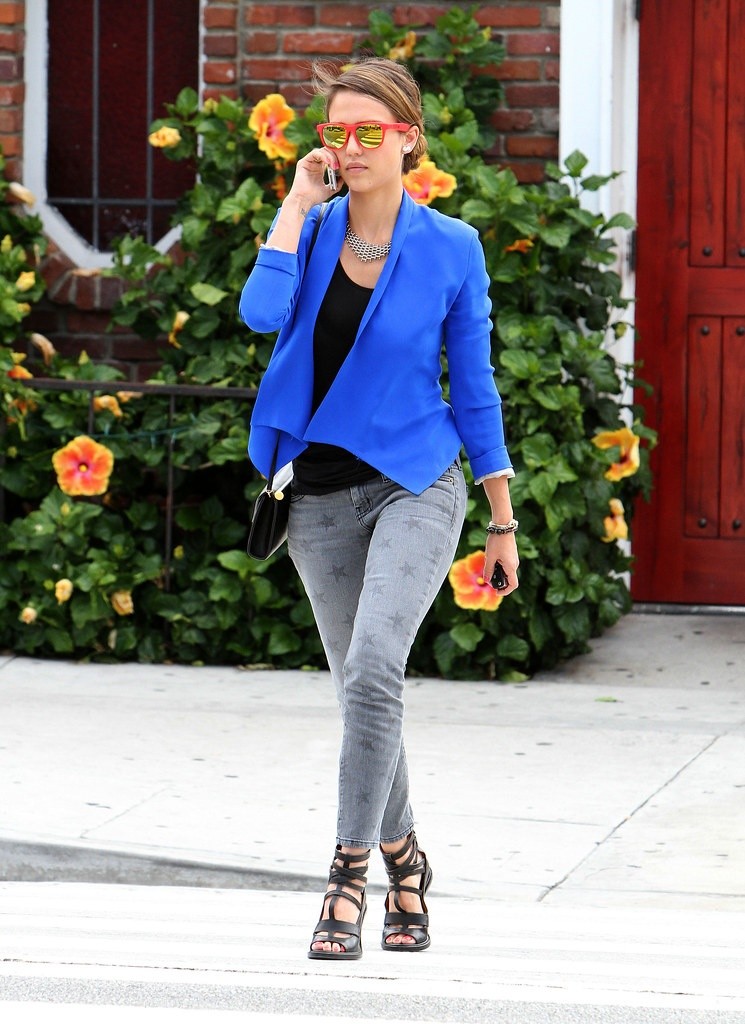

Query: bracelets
[486,519,520,536]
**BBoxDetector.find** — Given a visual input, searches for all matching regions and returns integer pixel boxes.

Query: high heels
[381,833,432,951]
[307,845,371,960]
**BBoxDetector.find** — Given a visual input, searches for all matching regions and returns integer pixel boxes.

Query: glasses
[316,121,412,151]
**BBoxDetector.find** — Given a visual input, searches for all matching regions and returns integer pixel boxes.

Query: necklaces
[344,217,392,263]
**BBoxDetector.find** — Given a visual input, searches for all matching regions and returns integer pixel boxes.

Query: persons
[237,55,522,960]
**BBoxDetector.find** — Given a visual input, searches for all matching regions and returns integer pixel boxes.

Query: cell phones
[327,164,337,190]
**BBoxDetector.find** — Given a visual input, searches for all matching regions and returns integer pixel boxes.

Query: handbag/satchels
[246,460,293,561]
